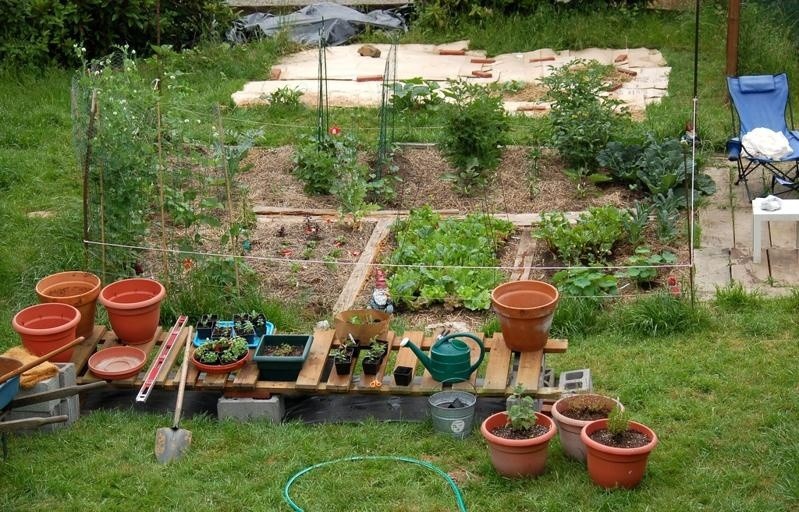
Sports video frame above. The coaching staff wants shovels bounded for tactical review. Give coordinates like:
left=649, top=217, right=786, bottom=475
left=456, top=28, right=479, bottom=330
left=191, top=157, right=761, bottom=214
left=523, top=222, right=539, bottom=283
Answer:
left=154, top=325, right=193, bottom=466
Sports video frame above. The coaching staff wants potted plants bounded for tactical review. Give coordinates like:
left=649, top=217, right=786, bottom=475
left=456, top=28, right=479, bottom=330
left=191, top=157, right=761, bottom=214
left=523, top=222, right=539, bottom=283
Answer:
left=479, top=381, right=558, bottom=479
left=190, top=335, right=250, bottom=374
left=552, top=382, right=625, bottom=462
left=580, top=405, right=658, bottom=489
left=253, top=333, right=314, bottom=381
left=195, top=312, right=266, bottom=344
left=333, top=336, right=387, bottom=375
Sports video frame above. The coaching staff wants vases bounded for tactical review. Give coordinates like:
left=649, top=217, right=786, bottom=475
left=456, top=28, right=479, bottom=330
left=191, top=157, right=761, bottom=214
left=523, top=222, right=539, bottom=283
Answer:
left=490, top=279, right=559, bottom=352
left=97, top=278, right=166, bottom=346
left=11, top=303, right=81, bottom=363
left=36, top=270, right=102, bottom=342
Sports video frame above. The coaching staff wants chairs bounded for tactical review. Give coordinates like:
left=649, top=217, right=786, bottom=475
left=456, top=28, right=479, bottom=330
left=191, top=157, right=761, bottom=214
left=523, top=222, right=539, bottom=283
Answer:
left=726, top=72, right=799, bottom=203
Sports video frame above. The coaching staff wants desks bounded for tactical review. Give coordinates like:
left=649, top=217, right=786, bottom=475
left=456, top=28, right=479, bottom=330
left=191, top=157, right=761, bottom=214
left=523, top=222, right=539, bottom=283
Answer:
left=751, top=198, right=799, bottom=264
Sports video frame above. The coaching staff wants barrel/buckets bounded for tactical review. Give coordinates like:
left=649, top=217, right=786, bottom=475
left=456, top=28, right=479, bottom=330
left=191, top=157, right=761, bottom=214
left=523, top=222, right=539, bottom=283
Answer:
left=427, top=378, right=477, bottom=441
